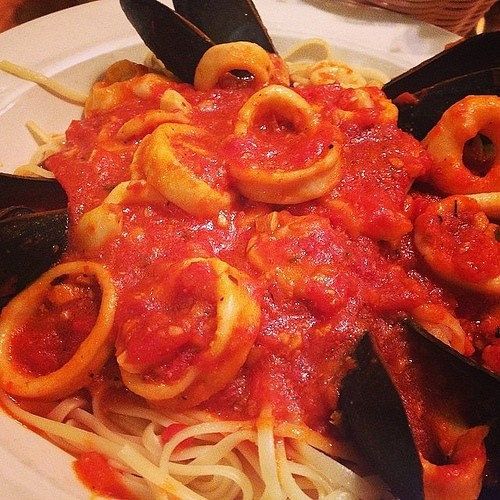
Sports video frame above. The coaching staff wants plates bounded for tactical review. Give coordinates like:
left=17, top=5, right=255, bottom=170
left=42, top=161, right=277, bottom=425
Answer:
left=0, top=0, right=500, bottom=500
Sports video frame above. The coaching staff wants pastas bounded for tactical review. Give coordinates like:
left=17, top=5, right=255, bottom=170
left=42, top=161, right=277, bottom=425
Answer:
left=0, top=36, right=500, bottom=500
